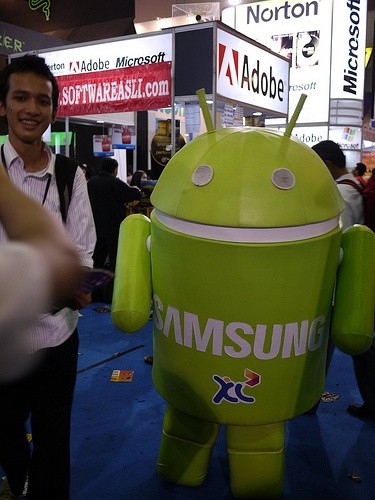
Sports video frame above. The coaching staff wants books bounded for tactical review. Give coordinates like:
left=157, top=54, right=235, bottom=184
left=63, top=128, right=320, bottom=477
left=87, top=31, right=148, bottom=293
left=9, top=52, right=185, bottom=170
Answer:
left=48, top=266, right=115, bottom=315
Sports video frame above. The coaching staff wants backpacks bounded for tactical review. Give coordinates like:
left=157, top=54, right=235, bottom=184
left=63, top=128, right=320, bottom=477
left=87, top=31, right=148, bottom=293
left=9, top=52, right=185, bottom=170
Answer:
left=337, top=179, right=375, bottom=233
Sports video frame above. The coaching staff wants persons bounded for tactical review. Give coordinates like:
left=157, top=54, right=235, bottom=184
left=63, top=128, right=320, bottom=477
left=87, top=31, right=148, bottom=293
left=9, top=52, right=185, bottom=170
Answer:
left=0, top=54, right=97, bottom=500
left=0, top=160, right=83, bottom=341
left=80, top=155, right=153, bottom=268
left=303, top=140, right=375, bottom=420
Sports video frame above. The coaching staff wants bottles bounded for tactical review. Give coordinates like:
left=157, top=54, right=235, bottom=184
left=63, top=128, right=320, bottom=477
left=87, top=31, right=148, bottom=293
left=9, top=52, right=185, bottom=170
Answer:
left=102, top=137, right=110, bottom=152
left=122, top=127, right=131, bottom=144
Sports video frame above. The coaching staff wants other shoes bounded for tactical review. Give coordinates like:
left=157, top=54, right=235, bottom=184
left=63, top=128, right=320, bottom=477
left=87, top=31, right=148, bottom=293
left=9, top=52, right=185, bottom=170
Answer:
left=143, top=355, right=153, bottom=365
left=348, top=403, right=375, bottom=420
